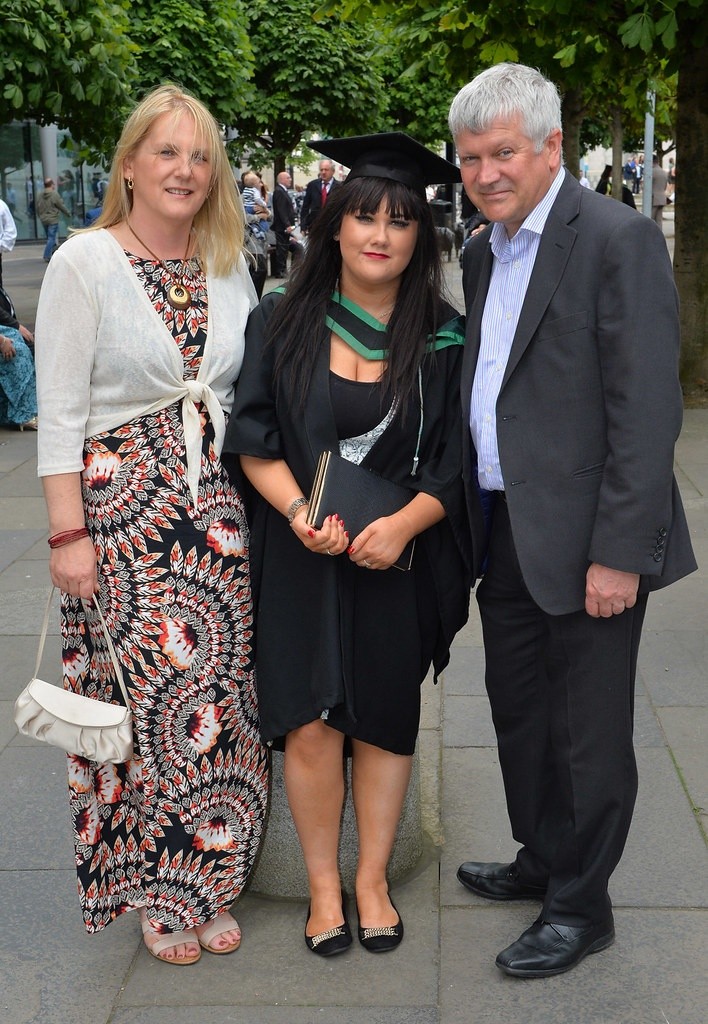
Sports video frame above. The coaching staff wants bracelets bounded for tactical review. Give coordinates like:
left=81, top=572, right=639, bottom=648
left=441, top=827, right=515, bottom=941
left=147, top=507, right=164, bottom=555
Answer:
left=287, top=498, right=310, bottom=523
left=48, top=526, right=91, bottom=550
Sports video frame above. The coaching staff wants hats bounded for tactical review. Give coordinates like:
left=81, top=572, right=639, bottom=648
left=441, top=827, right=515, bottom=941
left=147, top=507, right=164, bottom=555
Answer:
left=306, top=131, right=463, bottom=200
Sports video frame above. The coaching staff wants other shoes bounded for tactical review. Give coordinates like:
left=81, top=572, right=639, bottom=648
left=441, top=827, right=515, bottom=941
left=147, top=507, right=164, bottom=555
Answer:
left=275, top=273, right=287, bottom=278
left=355, top=892, right=404, bottom=953
left=44, top=257, right=51, bottom=263
left=304, top=888, right=353, bottom=957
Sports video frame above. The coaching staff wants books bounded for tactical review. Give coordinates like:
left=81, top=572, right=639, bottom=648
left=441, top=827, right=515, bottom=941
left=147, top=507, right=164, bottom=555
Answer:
left=305, top=451, right=417, bottom=574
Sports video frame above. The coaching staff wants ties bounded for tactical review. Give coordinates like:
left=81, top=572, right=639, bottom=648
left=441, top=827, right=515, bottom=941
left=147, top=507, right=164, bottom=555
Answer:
left=321, top=183, right=329, bottom=209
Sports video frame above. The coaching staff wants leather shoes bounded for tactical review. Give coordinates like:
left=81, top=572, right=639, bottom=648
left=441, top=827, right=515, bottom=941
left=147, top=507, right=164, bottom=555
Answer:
left=495, top=916, right=615, bottom=977
left=456, top=861, right=548, bottom=901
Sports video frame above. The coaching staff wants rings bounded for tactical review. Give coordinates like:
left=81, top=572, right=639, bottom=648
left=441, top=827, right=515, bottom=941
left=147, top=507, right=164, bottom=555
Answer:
left=326, top=549, right=335, bottom=556
left=363, top=559, right=371, bottom=567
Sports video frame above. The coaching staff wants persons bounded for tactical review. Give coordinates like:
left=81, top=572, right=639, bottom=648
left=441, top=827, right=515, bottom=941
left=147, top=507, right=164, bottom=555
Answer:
left=462, top=187, right=490, bottom=252
left=238, top=160, right=343, bottom=302
left=0, top=175, right=34, bottom=431
left=578, top=151, right=675, bottom=233
left=219, top=132, right=476, bottom=959
left=446, top=62, right=699, bottom=981
left=35, top=85, right=274, bottom=966
left=36, top=167, right=106, bottom=263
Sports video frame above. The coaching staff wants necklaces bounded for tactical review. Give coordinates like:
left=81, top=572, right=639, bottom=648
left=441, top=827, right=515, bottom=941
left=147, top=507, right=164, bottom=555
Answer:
left=358, top=306, right=394, bottom=321
left=122, top=220, right=198, bottom=310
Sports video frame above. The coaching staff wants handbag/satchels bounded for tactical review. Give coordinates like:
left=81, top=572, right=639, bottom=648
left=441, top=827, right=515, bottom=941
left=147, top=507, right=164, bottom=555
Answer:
left=14, top=585, right=134, bottom=764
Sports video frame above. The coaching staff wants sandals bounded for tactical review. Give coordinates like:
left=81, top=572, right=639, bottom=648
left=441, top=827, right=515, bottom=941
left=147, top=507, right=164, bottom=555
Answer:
left=142, top=911, right=242, bottom=965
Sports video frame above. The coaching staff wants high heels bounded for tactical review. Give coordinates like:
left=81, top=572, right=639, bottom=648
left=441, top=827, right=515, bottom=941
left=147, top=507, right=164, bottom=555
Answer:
left=19, top=417, right=37, bottom=431
